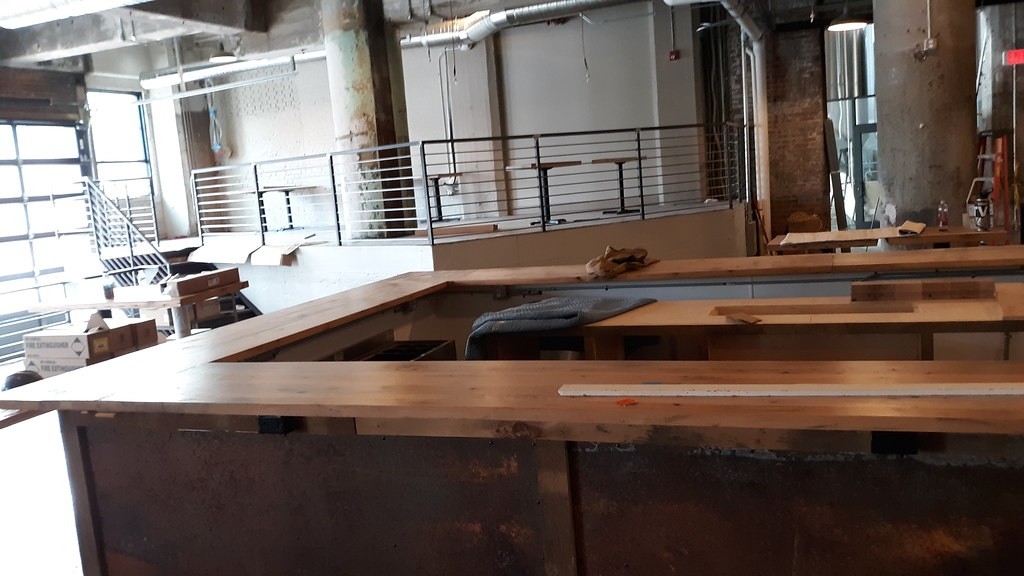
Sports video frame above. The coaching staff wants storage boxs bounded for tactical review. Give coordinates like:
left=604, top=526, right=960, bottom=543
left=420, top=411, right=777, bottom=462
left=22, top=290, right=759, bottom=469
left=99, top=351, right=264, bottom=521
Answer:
left=22, top=295, right=222, bottom=378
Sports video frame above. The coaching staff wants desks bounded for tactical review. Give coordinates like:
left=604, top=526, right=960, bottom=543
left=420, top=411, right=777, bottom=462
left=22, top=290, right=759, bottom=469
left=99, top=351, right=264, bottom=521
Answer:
left=591, top=155, right=647, bottom=215
left=29, top=279, right=250, bottom=347
left=506, top=160, right=582, bottom=225
left=263, top=185, right=316, bottom=231
left=423, top=173, right=462, bottom=221
left=767, top=226, right=1007, bottom=254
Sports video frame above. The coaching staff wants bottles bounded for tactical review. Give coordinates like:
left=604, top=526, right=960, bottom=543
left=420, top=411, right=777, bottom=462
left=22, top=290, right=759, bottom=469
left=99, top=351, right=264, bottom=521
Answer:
left=937, top=200, right=950, bottom=231
left=974, top=187, right=994, bottom=232
